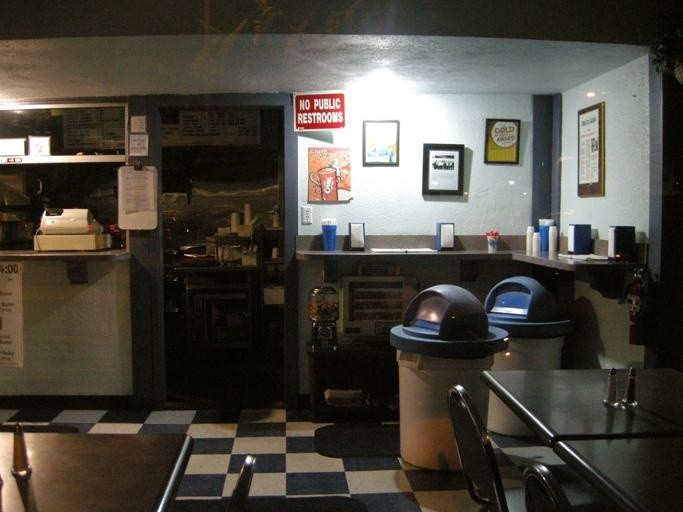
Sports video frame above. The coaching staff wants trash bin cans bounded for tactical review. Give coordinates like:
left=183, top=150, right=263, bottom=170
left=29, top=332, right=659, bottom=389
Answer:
left=390, top=285, right=509, bottom=472
left=484, top=274, right=574, bottom=440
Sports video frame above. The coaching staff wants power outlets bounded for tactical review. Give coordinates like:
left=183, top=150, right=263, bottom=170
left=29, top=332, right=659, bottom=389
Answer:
left=300, top=205, right=312, bottom=224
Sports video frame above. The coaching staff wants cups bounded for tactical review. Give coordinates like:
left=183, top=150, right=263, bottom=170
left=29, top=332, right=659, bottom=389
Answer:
left=538, top=224, right=548, bottom=251
left=487, top=236, right=498, bottom=250
left=310, top=167, right=338, bottom=200
left=322, top=224, right=337, bottom=251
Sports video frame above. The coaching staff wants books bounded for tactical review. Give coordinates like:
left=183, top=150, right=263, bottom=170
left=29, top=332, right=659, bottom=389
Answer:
left=556, top=252, right=608, bottom=261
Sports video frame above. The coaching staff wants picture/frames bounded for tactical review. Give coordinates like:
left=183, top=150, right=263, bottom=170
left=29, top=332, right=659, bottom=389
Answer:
left=361, top=119, right=520, bottom=196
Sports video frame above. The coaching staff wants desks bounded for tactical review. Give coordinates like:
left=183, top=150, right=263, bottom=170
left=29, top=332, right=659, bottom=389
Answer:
left=481, top=366, right=682, bottom=512
left=0, top=431, right=194, bottom=512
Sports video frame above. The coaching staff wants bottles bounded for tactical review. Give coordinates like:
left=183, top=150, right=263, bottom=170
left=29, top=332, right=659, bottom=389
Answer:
left=271, top=247, right=280, bottom=258
left=243, top=203, right=252, bottom=226
left=525, top=225, right=541, bottom=251
left=231, top=212, right=240, bottom=233
left=272, top=204, right=280, bottom=228
left=602, top=366, right=637, bottom=407
left=547, top=225, right=558, bottom=251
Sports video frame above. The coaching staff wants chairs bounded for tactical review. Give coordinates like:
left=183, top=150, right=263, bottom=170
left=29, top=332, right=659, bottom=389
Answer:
left=446, top=383, right=509, bottom=511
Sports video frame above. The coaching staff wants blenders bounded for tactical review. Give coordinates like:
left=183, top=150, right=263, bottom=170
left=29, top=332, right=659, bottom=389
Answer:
left=308, top=287, right=339, bottom=353
